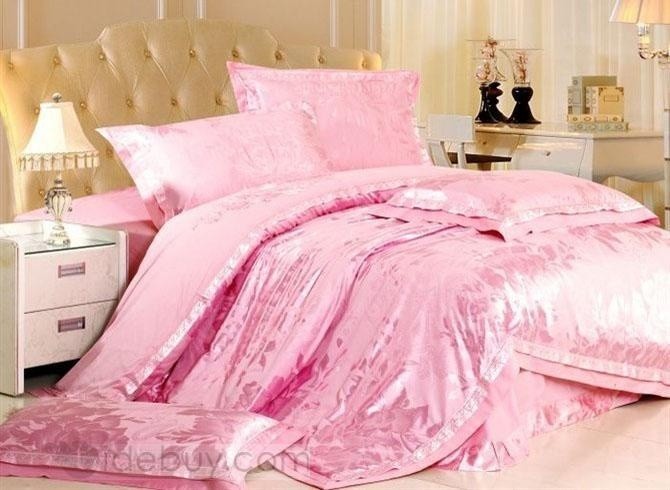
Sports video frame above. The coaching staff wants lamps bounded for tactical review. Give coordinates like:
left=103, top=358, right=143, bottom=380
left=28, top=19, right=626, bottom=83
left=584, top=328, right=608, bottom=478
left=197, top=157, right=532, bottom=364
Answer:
left=609, top=1, right=670, bottom=64
left=17, top=93, right=100, bottom=246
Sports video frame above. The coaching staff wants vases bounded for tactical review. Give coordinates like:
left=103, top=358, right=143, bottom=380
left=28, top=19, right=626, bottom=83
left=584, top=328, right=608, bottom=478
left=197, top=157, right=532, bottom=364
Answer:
left=465, top=33, right=545, bottom=124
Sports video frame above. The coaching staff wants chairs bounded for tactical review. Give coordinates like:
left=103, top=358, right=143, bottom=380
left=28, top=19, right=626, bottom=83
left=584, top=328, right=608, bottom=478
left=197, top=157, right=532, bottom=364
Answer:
left=426, top=114, right=512, bottom=170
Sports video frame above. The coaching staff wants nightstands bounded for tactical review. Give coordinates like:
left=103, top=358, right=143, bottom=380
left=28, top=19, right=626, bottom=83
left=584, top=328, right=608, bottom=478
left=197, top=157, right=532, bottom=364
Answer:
left=0, top=221, right=129, bottom=396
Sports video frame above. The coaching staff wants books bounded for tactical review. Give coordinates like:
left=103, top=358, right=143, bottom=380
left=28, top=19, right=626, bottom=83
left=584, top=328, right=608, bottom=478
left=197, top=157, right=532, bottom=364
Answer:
left=566, top=74, right=630, bottom=134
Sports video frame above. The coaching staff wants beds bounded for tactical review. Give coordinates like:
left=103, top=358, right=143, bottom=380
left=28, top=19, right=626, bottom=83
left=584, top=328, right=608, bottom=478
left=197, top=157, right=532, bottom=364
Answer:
left=1, top=19, right=670, bottom=471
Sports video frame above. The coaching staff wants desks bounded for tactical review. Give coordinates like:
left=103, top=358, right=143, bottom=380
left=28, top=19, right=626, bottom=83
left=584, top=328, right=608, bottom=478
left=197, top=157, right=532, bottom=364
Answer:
left=447, top=124, right=665, bottom=230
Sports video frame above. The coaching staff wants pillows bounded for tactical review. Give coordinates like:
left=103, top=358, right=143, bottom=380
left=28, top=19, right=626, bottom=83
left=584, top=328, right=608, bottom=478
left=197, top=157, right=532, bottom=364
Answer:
left=226, top=61, right=435, bottom=172
left=0, top=399, right=305, bottom=490
left=94, top=108, right=339, bottom=230
left=367, top=170, right=661, bottom=243
